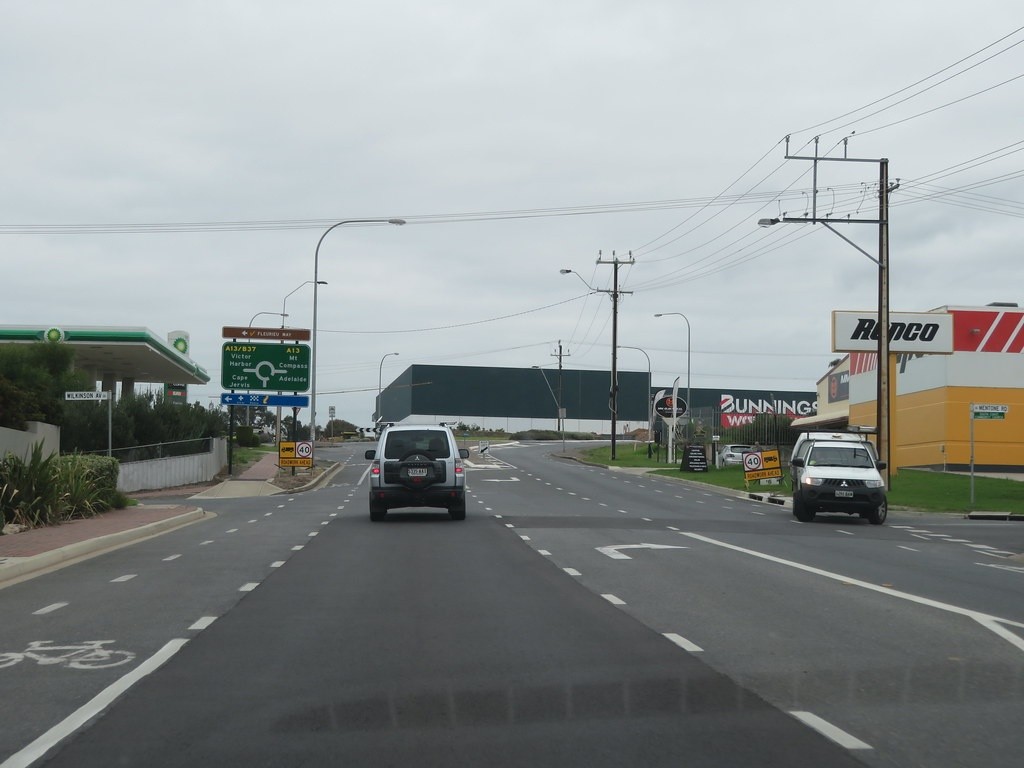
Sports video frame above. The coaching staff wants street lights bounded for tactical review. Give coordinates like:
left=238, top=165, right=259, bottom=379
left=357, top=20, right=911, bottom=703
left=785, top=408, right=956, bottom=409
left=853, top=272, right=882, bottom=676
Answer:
left=246, top=311, right=290, bottom=341
left=282, top=280, right=328, bottom=325
left=378, top=353, right=400, bottom=421
left=757, top=216, right=892, bottom=503
left=616, top=345, right=652, bottom=441
left=654, top=312, right=691, bottom=443
left=560, top=269, right=619, bottom=460
left=308, top=219, right=407, bottom=443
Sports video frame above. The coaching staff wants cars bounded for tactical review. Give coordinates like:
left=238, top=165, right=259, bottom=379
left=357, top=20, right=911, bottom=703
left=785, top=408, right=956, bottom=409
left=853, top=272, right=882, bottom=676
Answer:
left=718, top=444, right=755, bottom=468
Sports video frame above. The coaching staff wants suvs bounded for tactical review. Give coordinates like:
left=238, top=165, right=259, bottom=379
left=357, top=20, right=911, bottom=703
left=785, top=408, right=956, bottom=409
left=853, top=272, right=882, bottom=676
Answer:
left=364, top=423, right=470, bottom=521
left=787, top=430, right=888, bottom=526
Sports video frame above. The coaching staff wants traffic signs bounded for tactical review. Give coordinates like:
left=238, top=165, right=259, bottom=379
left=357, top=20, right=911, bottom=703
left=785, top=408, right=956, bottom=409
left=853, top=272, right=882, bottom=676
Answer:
left=221, top=342, right=310, bottom=391
left=220, top=392, right=310, bottom=407
left=222, top=326, right=311, bottom=341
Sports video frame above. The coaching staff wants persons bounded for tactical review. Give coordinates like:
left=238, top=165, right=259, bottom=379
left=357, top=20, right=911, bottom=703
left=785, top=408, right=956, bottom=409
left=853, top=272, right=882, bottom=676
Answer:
left=810, top=450, right=832, bottom=465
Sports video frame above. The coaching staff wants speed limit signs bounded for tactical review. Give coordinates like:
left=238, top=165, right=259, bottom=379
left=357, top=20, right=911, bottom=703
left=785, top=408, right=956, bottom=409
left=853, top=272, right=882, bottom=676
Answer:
left=296, top=441, right=313, bottom=457
left=742, top=452, right=762, bottom=471
